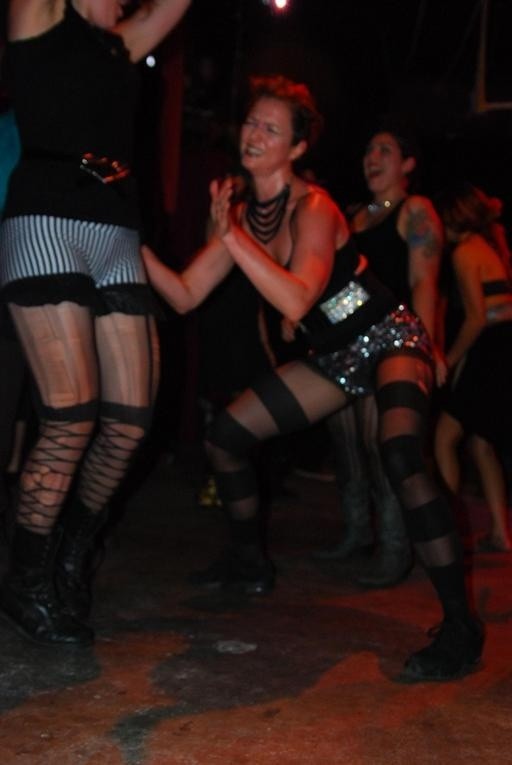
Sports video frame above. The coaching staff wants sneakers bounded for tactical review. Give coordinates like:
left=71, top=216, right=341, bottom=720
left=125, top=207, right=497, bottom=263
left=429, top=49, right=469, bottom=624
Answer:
left=188, top=554, right=275, bottom=594
left=405, top=616, right=484, bottom=680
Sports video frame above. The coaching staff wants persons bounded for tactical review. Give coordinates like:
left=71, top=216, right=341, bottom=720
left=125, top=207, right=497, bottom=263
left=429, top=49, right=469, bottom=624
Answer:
left=278, top=120, right=445, bottom=593
left=137, top=72, right=488, bottom=684
left=0, top=0, right=193, bottom=653
left=432, top=178, right=512, bottom=559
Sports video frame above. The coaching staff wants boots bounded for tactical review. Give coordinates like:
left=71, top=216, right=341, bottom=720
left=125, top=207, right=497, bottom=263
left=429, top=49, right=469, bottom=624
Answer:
left=340, top=474, right=413, bottom=590
left=0, top=499, right=110, bottom=649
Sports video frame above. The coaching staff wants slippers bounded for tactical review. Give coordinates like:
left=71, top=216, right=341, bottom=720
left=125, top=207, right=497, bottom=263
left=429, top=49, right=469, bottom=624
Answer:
left=460, top=536, right=512, bottom=555
